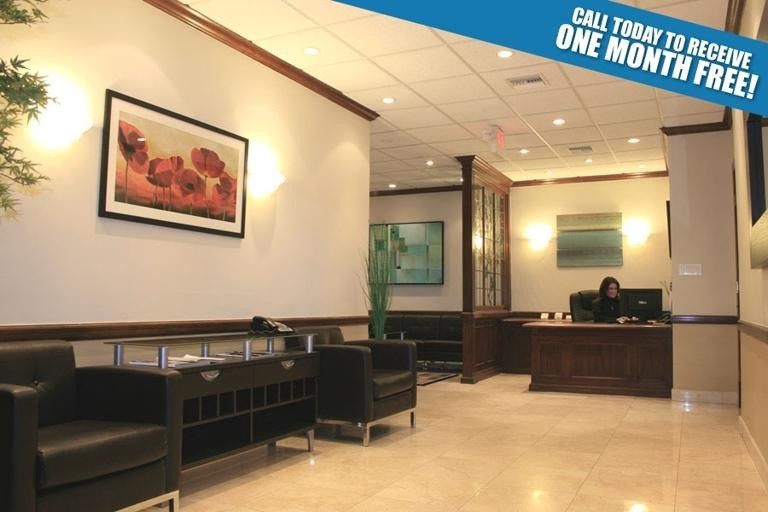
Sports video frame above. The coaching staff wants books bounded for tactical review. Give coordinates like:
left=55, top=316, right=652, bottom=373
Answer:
left=128, top=351, right=283, bottom=369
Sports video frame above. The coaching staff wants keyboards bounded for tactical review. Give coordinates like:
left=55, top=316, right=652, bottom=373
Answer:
left=608, top=320, right=639, bottom=323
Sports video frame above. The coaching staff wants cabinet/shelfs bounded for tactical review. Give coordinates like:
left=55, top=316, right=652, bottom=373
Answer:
left=109, top=337, right=324, bottom=510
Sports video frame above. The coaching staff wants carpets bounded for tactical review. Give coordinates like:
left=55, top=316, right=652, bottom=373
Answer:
left=417, top=371, right=458, bottom=386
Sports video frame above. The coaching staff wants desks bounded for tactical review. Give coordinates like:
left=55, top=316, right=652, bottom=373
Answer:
left=521, top=320, right=673, bottom=397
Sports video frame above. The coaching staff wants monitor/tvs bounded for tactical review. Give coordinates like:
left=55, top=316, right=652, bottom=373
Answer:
left=619, top=289, right=662, bottom=324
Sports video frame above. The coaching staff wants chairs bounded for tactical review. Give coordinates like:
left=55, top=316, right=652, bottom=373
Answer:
left=287, top=325, right=419, bottom=447
left=0, top=342, right=183, bottom=512
left=570, top=289, right=601, bottom=324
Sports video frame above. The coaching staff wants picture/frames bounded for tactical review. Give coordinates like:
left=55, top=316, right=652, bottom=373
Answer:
left=97, top=90, right=253, bottom=239
left=369, top=222, right=445, bottom=287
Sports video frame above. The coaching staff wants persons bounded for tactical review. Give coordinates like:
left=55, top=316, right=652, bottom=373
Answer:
left=592, top=277, right=639, bottom=324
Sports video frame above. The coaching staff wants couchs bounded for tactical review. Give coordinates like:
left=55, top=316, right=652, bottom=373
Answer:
left=370, top=313, right=464, bottom=371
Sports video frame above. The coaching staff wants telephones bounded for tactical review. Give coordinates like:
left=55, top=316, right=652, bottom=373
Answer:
left=250, top=316, right=294, bottom=335
left=657, top=312, right=671, bottom=324
